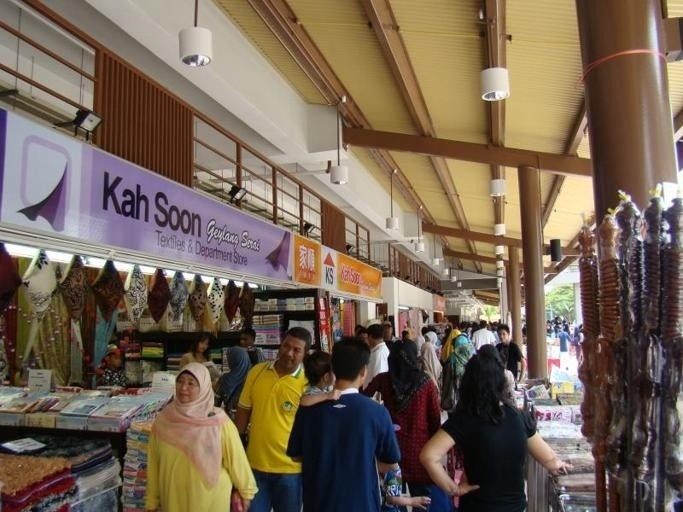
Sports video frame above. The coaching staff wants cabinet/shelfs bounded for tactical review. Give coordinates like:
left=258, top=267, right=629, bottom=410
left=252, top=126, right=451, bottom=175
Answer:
left=440, top=293, right=443, bottom=296
left=340, top=298, right=359, bottom=338
left=119, top=287, right=334, bottom=396
left=0, top=383, right=163, bottom=512
left=512, top=376, right=595, bottom=512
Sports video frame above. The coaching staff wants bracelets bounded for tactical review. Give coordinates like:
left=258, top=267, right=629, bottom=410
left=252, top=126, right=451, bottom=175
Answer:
left=447, top=485, right=458, bottom=495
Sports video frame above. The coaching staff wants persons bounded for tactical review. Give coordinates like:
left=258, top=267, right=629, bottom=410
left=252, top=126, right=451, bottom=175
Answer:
left=494, top=325, right=525, bottom=391
left=574, top=324, right=585, bottom=365
left=379, top=464, right=431, bottom=512
left=420, top=321, right=479, bottom=339
left=235, top=327, right=311, bottom=512
left=298, top=350, right=341, bottom=406
left=417, top=343, right=443, bottom=410
left=471, top=320, right=497, bottom=355
left=360, top=339, right=442, bottom=512
left=487, top=319, right=503, bottom=331
left=358, top=328, right=369, bottom=344
left=237, top=328, right=267, bottom=368
left=180, top=335, right=210, bottom=371
left=544, top=317, right=570, bottom=336
left=213, top=347, right=253, bottom=421
left=400, top=330, right=419, bottom=355
left=442, top=329, right=462, bottom=363
left=362, top=324, right=390, bottom=391
left=354, top=324, right=363, bottom=334
left=420, top=356, right=575, bottom=512
left=83, top=349, right=130, bottom=386
left=440, top=334, right=475, bottom=418
left=286, top=336, right=401, bottom=512
left=381, top=320, right=400, bottom=350
left=425, top=332, right=440, bottom=359
left=559, top=329, right=572, bottom=370
left=144, top=363, right=260, bottom=512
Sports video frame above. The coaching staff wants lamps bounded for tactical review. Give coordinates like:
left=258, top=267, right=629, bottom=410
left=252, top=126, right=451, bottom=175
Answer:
left=437, top=291, right=441, bottom=294
left=347, top=244, right=352, bottom=252
left=229, top=186, right=247, bottom=201
left=494, top=197, right=505, bottom=235
left=497, top=283, right=502, bottom=288
left=432, top=233, right=440, bottom=265
left=386, top=169, right=398, bottom=229
left=303, top=223, right=315, bottom=234
left=405, top=275, right=411, bottom=280
left=496, top=255, right=504, bottom=269
left=415, top=281, right=420, bottom=284
left=330, top=102, right=349, bottom=184
left=413, top=203, right=425, bottom=252
left=432, top=290, right=437, bottom=293
left=179, top=0, right=214, bottom=67
left=75, top=110, right=103, bottom=132
left=443, top=253, right=449, bottom=276
left=497, top=277, right=503, bottom=282
left=427, top=286, right=432, bottom=291
left=457, top=274, right=462, bottom=288
left=451, top=265, right=456, bottom=281
left=479, top=18, right=509, bottom=100
left=496, top=236, right=504, bottom=254
left=496, top=269, right=504, bottom=276
left=491, top=140, right=506, bottom=196
